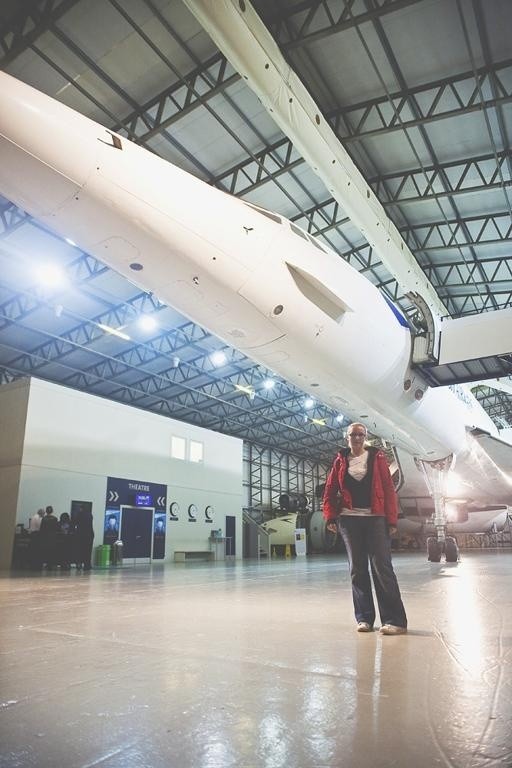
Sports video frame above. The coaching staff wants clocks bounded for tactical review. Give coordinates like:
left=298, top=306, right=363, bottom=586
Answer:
left=188, top=503, right=197, bottom=518
left=205, top=505, right=215, bottom=519
left=169, top=502, right=181, bottom=516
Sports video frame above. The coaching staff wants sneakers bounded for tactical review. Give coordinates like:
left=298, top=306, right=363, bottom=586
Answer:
left=378, top=623, right=407, bottom=635
left=356, top=620, right=373, bottom=632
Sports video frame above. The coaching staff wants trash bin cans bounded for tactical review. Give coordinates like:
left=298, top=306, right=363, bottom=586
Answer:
left=97, top=544, right=111, bottom=568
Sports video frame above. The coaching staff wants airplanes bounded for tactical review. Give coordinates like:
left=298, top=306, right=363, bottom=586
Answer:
left=0, top=73, right=512, bottom=564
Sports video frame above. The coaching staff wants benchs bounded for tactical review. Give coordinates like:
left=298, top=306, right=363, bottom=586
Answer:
left=174, top=550, right=216, bottom=562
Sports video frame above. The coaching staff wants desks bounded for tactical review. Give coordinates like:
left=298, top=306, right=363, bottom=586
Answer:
left=209, top=536, right=233, bottom=561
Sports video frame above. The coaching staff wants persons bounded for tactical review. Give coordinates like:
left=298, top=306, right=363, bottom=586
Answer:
left=323, top=422, right=408, bottom=635
left=103, top=514, right=120, bottom=545
left=151, top=518, right=167, bottom=559
left=26, top=504, right=95, bottom=569
